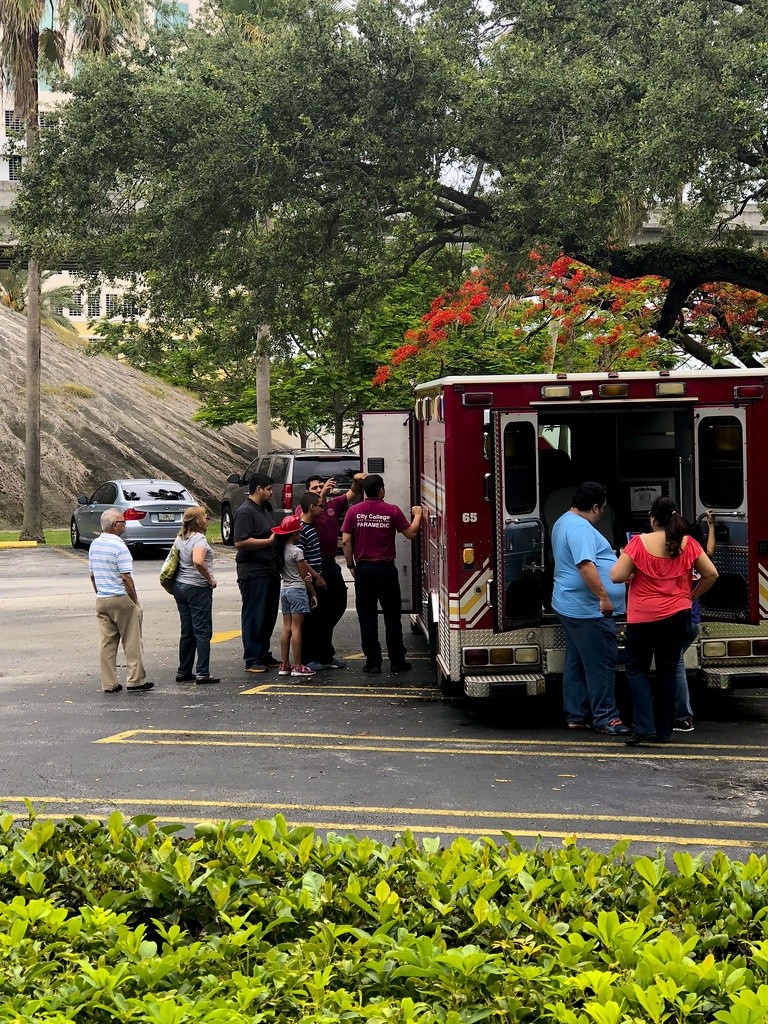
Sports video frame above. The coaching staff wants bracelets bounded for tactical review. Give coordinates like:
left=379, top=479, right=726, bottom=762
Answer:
left=347, top=562, right=355, bottom=569
left=316, top=574, right=318, bottom=578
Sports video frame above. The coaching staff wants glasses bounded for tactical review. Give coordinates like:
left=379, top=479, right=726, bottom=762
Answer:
left=111, top=521, right=126, bottom=526
left=205, top=516, right=210, bottom=520
left=309, top=504, right=325, bottom=509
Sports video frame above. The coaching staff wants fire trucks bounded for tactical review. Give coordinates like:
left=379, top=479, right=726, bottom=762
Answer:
left=357, top=367, right=768, bottom=719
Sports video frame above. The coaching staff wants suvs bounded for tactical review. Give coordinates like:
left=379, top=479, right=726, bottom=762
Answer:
left=220, top=448, right=361, bottom=545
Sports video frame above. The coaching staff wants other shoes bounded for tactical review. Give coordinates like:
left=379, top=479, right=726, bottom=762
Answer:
left=306, top=658, right=347, bottom=670
left=244, top=663, right=267, bottom=672
left=624, top=733, right=656, bottom=745
left=127, top=681, right=154, bottom=691
left=105, top=684, right=123, bottom=693
left=362, top=664, right=382, bottom=674
left=175, top=673, right=196, bottom=682
left=267, top=660, right=283, bottom=666
left=196, top=675, right=220, bottom=684
left=390, top=662, right=413, bottom=672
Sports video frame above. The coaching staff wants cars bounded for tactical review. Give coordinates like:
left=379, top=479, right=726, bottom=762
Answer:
left=70, top=478, right=199, bottom=555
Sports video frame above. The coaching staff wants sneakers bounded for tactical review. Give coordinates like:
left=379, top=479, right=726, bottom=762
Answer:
left=278, top=664, right=291, bottom=675
left=568, top=717, right=593, bottom=729
left=290, top=664, right=316, bottom=676
left=672, top=719, right=694, bottom=732
left=597, top=717, right=630, bottom=734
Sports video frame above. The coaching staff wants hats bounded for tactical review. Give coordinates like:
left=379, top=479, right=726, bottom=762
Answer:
left=271, top=516, right=304, bottom=534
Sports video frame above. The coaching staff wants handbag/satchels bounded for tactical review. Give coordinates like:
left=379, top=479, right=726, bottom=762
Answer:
left=160, top=547, right=178, bottom=594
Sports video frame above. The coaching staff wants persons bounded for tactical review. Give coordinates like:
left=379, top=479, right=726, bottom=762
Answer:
left=296, top=492, right=328, bottom=672
left=163, top=507, right=224, bottom=685
left=340, top=475, right=421, bottom=673
left=550, top=482, right=631, bottom=737
left=271, top=515, right=319, bottom=677
left=669, top=509, right=718, bottom=737
left=295, top=471, right=368, bottom=672
left=233, top=473, right=284, bottom=674
left=611, top=496, right=717, bottom=747
left=88, top=508, right=155, bottom=694
